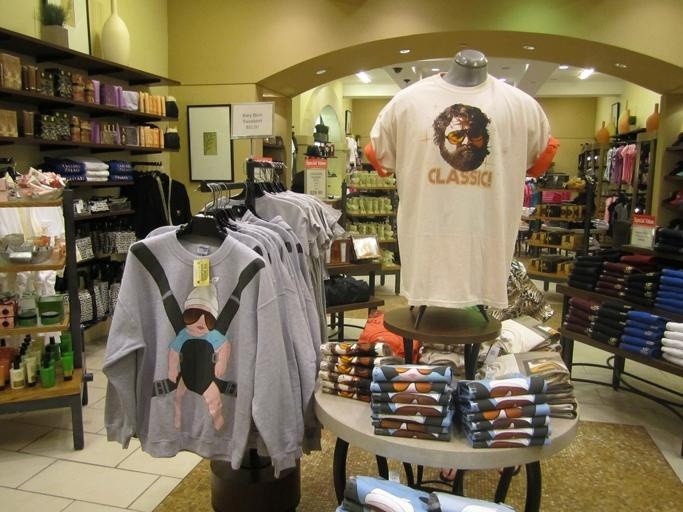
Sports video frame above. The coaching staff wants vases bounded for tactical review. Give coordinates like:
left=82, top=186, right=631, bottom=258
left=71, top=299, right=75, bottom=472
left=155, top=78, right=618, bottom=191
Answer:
left=645, top=103, right=660, bottom=134
left=596, top=121, right=609, bottom=143
left=100, top=1, right=132, bottom=67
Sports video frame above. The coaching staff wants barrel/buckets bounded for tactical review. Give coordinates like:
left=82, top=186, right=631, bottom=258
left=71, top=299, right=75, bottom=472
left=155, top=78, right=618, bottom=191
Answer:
left=116, top=121, right=121, bottom=144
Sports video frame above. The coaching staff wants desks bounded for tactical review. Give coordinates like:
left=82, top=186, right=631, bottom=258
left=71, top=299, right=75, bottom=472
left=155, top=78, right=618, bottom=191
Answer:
left=310, top=352, right=581, bottom=511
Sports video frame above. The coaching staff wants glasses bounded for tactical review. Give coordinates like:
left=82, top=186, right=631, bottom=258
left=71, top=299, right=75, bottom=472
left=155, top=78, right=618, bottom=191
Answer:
left=182, top=309, right=216, bottom=331
left=444, top=125, right=488, bottom=146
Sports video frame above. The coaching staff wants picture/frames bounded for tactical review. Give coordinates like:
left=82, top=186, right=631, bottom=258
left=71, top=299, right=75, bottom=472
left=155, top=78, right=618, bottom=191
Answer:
left=186, top=103, right=235, bottom=184
left=611, top=102, right=619, bottom=134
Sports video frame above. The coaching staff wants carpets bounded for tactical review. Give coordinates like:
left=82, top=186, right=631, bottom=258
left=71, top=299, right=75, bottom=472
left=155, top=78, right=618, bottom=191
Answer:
left=151, top=421, right=682, bottom=511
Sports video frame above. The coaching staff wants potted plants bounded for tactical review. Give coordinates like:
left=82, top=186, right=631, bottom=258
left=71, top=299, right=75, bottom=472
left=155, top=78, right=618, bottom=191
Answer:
left=40, top=4, right=69, bottom=48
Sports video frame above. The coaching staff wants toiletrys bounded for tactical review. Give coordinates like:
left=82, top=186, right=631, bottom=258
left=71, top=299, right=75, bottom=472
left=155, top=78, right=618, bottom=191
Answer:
left=92, top=79, right=101, bottom=104
left=21, top=63, right=40, bottom=91
left=60, top=330, right=75, bottom=381
left=95, top=122, right=101, bottom=144
left=113, top=85, right=127, bottom=107
left=139, top=91, right=167, bottom=150
left=0, top=332, right=60, bottom=389
left=22, top=108, right=35, bottom=136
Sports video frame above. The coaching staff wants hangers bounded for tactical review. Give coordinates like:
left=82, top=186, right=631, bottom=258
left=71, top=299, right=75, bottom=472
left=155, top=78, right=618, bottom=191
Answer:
left=177, top=161, right=287, bottom=241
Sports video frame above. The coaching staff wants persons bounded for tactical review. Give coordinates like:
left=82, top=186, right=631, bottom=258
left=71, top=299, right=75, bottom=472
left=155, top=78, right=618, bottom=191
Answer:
left=435, top=103, right=490, bottom=172
left=166, top=283, right=230, bottom=432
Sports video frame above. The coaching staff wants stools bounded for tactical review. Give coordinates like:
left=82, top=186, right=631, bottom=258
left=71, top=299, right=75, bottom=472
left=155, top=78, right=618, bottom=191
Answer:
left=382, top=307, right=504, bottom=381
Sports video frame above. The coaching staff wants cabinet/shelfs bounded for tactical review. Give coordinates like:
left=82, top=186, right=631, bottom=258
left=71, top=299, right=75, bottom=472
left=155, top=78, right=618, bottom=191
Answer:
left=1, top=186, right=95, bottom=450
left=514, top=127, right=683, bottom=449
left=1, top=29, right=184, bottom=342
left=325, top=181, right=403, bottom=344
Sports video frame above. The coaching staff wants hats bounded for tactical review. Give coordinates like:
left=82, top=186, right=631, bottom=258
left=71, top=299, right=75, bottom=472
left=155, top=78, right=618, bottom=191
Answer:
left=183, top=283, right=218, bottom=320
